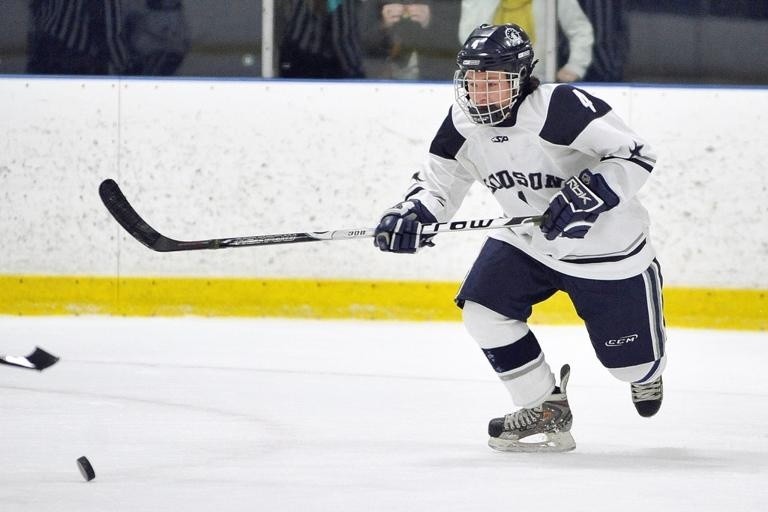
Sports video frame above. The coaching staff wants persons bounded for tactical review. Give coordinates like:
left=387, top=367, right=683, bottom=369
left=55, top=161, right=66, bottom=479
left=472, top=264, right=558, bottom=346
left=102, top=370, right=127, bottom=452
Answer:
left=274, top=1, right=362, bottom=82
left=372, top=22, right=666, bottom=442
left=557, top=1, right=637, bottom=86
left=457, top=1, right=591, bottom=84
left=360, top=1, right=460, bottom=77
left=25, top=0, right=193, bottom=77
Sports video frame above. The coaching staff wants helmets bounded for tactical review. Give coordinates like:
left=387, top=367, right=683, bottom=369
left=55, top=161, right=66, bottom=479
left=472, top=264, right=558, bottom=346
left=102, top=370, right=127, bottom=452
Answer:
left=453, top=23, right=540, bottom=127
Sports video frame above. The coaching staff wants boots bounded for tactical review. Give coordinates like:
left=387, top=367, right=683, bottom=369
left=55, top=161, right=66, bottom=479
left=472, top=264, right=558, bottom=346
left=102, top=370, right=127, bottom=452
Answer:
left=631, top=374, right=663, bottom=418
left=488, top=364, right=573, bottom=441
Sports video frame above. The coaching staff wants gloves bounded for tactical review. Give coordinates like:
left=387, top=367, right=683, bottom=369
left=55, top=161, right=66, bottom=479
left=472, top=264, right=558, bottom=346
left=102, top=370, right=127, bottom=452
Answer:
left=373, top=199, right=439, bottom=254
left=540, top=169, right=620, bottom=240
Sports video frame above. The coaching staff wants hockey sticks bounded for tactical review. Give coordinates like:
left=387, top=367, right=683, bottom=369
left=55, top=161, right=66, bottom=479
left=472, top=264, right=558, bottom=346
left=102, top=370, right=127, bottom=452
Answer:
left=96, top=178, right=547, bottom=253
